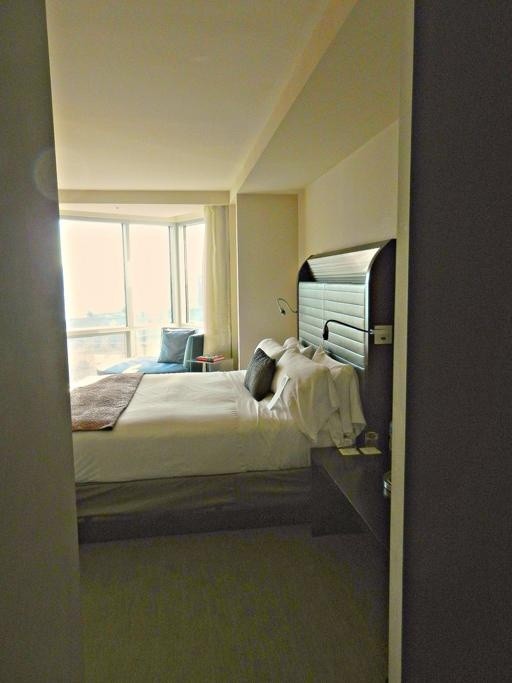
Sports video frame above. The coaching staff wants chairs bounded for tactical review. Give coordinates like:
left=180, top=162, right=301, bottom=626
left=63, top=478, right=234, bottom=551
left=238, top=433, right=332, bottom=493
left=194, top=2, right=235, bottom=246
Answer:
left=96, top=333, right=203, bottom=373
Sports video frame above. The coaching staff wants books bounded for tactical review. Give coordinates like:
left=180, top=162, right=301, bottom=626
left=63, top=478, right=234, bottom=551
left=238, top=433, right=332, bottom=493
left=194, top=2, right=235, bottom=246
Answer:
left=196, top=352, right=225, bottom=361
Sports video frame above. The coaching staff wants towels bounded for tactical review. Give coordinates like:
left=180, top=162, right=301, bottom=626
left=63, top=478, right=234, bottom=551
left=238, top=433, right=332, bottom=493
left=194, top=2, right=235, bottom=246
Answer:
left=69, top=373, right=143, bottom=431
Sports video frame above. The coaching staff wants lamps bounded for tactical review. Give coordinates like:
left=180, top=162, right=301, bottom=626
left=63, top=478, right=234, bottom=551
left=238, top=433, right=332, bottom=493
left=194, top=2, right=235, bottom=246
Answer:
left=276, top=295, right=392, bottom=346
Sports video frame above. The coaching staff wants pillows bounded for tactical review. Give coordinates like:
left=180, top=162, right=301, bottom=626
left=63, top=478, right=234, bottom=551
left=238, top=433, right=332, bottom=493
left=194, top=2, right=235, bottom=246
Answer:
left=156, top=326, right=195, bottom=364
left=243, top=335, right=367, bottom=446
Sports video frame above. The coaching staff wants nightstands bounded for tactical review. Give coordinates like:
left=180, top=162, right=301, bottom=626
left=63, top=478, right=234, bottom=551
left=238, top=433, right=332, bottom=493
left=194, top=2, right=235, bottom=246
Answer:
left=311, top=446, right=389, bottom=552
left=188, top=358, right=233, bottom=372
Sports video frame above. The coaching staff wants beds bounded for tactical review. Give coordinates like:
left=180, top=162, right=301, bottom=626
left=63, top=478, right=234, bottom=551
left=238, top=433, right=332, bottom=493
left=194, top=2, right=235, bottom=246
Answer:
left=68, top=237, right=395, bottom=539
